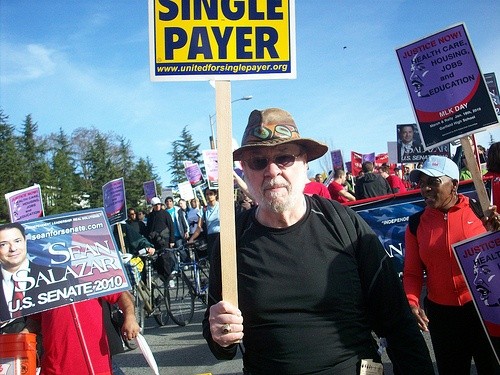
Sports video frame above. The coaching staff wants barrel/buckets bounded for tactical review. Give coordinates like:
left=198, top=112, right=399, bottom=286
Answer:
left=0, top=333, right=38, bottom=375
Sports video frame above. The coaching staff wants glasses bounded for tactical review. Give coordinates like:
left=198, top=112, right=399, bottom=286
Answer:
left=244, top=152, right=301, bottom=171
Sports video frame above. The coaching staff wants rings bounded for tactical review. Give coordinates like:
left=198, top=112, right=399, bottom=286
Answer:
left=494, top=217, right=497, bottom=220
left=221, top=323, right=232, bottom=334
left=123, top=332, right=127, bottom=337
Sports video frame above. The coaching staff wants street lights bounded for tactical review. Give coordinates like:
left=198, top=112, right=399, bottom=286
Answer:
left=209, top=96, right=253, bottom=148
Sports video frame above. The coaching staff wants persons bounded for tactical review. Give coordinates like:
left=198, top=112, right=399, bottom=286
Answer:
left=201, top=112, right=435, bottom=375
left=112, top=187, right=257, bottom=288
left=403, top=155, right=500, bottom=375
left=0, top=222, right=88, bottom=322
left=26, top=292, right=140, bottom=375
left=398, top=125, right=424, bottom=163
left=303, top=142, right=500, bottom=215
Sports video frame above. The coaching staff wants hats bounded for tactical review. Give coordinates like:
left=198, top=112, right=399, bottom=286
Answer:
left=233, top=107, right=329, bottom=163
left=409, top=154, right=460, bottom=183
left=151, top=197, right=162, bottom=205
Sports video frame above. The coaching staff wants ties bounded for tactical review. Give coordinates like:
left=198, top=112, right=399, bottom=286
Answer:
left=11, top=274, right=24, bottom=318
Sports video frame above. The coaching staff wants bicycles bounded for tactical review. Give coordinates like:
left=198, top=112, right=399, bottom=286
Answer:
left=107, top=238, right=211, bottom=355
